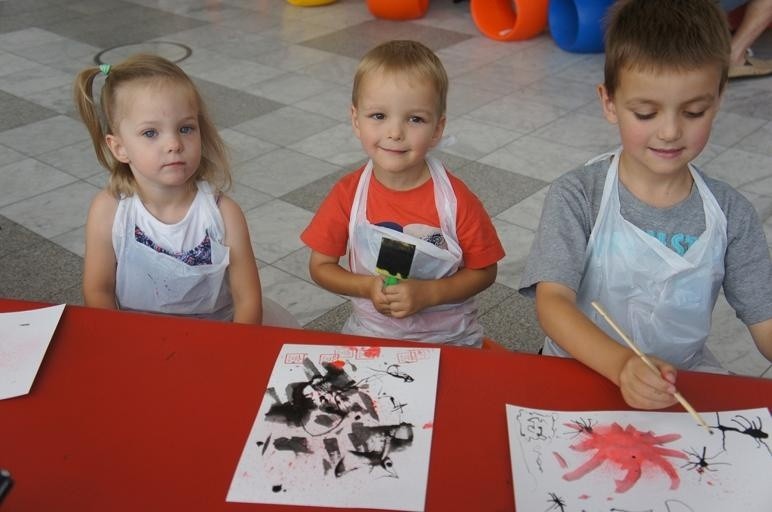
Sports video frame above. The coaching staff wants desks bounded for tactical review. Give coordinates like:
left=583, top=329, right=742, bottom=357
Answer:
left=0, top=296, right=772, bottom=512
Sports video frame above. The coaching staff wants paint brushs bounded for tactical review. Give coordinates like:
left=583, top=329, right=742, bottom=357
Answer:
left=591, top=301, right=714, bottom=435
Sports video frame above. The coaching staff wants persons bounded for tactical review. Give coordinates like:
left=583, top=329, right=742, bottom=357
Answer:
left=515, top=0, right=772, bottom=411
left=296, top=38, right=510, bottom=352
left=72, top=51, right=266, bottom=327
left=726, top=0, right=772, bottom=81
